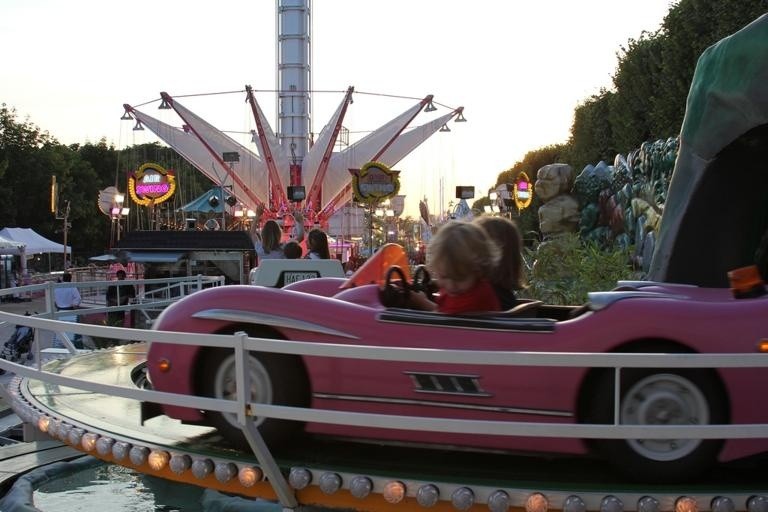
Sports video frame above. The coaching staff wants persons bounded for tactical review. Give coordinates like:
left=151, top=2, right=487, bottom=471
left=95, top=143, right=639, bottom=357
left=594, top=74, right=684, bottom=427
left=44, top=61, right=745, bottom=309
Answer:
left=304, top=229, right=330, bottom=259
left=408, top=220, right=501, bottom=316
left=249, top=202, right=285, bottom=267
left=474, top=215, right=524, bottom=312
left=53, top=272, right=83, bottom=350
left=283, top=240, right=303, bottom=260
left=103, top=270, right=136, bottom=346
left=341, top=247, right=350, bottom=275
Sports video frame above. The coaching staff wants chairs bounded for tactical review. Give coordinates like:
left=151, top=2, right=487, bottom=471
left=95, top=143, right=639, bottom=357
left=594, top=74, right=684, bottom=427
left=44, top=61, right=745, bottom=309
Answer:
left=485, top=295, right=547, bottom=319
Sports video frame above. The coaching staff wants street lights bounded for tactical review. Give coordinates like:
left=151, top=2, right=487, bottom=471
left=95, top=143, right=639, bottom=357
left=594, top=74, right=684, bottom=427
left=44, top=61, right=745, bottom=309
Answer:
left=111, top=192, right=130, bottom=240
